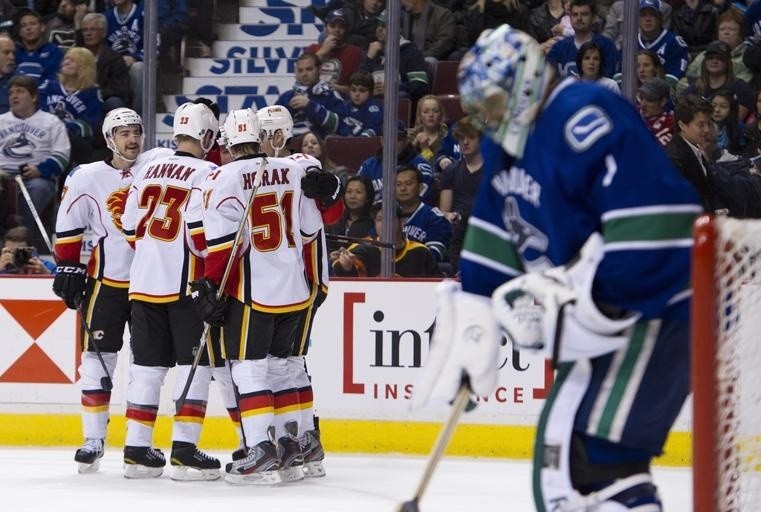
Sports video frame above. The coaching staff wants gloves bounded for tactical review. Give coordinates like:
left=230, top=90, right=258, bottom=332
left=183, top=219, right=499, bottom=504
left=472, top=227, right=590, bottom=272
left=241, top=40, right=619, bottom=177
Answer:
left=301, top=164, right=343, bottom=208
left=188, top=276, right=229, bottom=328
left=52, top=260, right=88, bottom=310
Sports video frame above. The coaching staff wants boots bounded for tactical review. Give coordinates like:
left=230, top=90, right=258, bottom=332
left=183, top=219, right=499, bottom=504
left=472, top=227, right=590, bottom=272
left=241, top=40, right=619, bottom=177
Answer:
left=226, top=415, right=325, bottom=475
left=75, top=418, right=109, bottom=464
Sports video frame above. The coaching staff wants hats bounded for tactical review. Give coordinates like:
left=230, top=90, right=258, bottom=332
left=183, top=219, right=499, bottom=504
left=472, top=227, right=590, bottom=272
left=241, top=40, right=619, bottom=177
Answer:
left=373, top=9, right=388, bottom=24
left=703, top=39, right=730, bottom=59
left=324, top=8, right=349, bottom=27
left=638, top=0, right=659, bottom=15
left=635, top=76, right=670, bottom=101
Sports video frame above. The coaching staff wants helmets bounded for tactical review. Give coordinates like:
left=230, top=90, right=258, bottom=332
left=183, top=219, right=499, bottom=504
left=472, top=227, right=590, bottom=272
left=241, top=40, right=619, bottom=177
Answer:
left=101, top=107, right=145, bottom=152
left=173, top=102, right=294, bottom=149
left=457, top=23, right=555, bottom=159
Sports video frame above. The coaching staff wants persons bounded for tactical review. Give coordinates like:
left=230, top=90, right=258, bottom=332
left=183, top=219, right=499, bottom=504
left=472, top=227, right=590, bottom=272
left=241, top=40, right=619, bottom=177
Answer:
left=53, top=102, right=345, bottom=486
left=1, top=1, right=240, bottom=274
left=406, top=23, right=741, bottom=512
left=274, top=1, right=761, bottom=281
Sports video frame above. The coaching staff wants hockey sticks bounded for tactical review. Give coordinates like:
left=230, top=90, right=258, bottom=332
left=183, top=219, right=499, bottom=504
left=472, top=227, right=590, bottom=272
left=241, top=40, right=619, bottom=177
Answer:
left=156, top=155, right=268, bottom=416
left=14, top=175, right=114, bottom=392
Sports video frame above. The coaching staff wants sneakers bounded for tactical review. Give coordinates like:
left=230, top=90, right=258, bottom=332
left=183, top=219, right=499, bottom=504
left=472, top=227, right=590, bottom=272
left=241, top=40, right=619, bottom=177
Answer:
left=124, top=443, right=167, bottom=467
left=170, top=442, right=221, bottom=469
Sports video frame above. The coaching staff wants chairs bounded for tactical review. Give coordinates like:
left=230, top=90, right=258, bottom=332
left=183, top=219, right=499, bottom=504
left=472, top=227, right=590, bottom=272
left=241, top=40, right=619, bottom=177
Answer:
left=376, top=98, right=411, bottom=132
left=432, top=61, right=462, bottom=95
left=437, top=95, right=464, bottom=126
left=158, top=36, right=186, bottom=82
left=322, top=135, right=383, bottom=184
left=424, top=57, right=438, bottom=95
